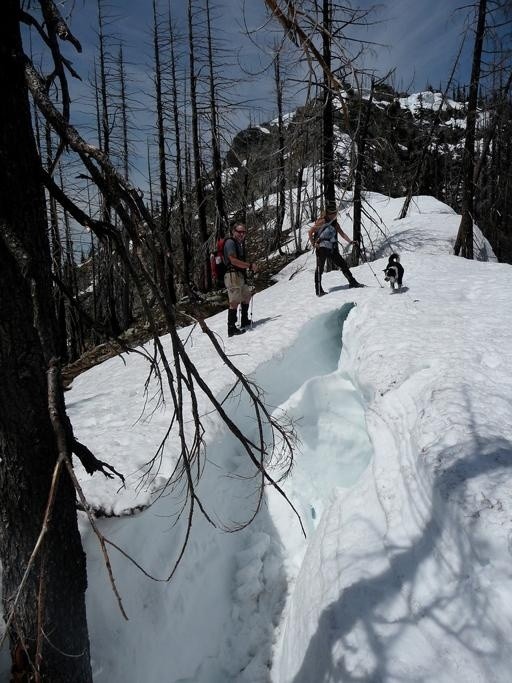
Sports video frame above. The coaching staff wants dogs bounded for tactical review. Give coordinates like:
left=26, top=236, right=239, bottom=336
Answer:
left=382, top=253, right=404, bottom=290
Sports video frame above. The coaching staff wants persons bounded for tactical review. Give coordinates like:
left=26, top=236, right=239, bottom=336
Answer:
left=221, top=220, right=261, bottom=337
left=308, top=204, right=366, bottom=297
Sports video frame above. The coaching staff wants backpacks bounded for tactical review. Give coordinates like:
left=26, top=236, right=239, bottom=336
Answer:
left=210, top=237, right=239, bottom=288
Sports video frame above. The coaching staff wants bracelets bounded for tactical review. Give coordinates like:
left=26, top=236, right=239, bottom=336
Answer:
left=249, top=263, right=252, bottom=269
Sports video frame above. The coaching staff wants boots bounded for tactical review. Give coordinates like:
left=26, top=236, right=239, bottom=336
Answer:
left=315, top=285, right=328, bottom=296
left=349, top=278, right=364, bottom=288
left=228, top=325, right=246, bottom=337
left=241, top=317, right=253, bottom=328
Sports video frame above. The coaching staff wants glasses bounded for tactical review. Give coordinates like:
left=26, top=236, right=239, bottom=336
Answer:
left=328, top=212, right=336, bottom=215
left=234, top=228, right=247, bottom=234
